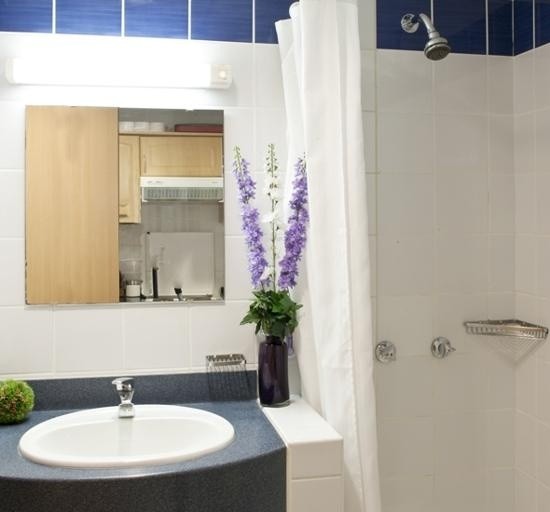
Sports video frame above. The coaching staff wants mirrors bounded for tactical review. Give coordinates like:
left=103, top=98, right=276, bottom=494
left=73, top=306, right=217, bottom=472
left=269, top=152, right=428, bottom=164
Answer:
left=118, top=107, right=227, bottom=303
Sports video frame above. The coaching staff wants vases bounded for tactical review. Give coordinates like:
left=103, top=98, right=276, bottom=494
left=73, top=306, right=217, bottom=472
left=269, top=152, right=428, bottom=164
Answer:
left=256, top=336, right=290, bottom=407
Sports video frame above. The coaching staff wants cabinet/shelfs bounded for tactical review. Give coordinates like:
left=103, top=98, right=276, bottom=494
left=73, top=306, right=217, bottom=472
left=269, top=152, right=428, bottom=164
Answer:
left=117, top=133, right=224, bottom=224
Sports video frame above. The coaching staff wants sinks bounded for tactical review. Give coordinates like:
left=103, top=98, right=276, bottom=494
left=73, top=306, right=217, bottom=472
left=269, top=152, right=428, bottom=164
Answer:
left=18, top=404, right=235, bottom=468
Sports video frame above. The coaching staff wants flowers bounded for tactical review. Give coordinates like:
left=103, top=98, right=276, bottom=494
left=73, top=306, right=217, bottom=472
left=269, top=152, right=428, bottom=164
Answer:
left=234, top=138, right=324, bottom=336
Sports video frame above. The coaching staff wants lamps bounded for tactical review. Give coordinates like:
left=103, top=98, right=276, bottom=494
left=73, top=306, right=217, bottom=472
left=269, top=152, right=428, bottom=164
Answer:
left=10, top=55, right=230, bottom=89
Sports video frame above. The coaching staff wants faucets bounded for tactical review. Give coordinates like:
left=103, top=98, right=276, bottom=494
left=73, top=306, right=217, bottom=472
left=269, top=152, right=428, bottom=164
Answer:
left=112, top=377, right=136, bottom=416
